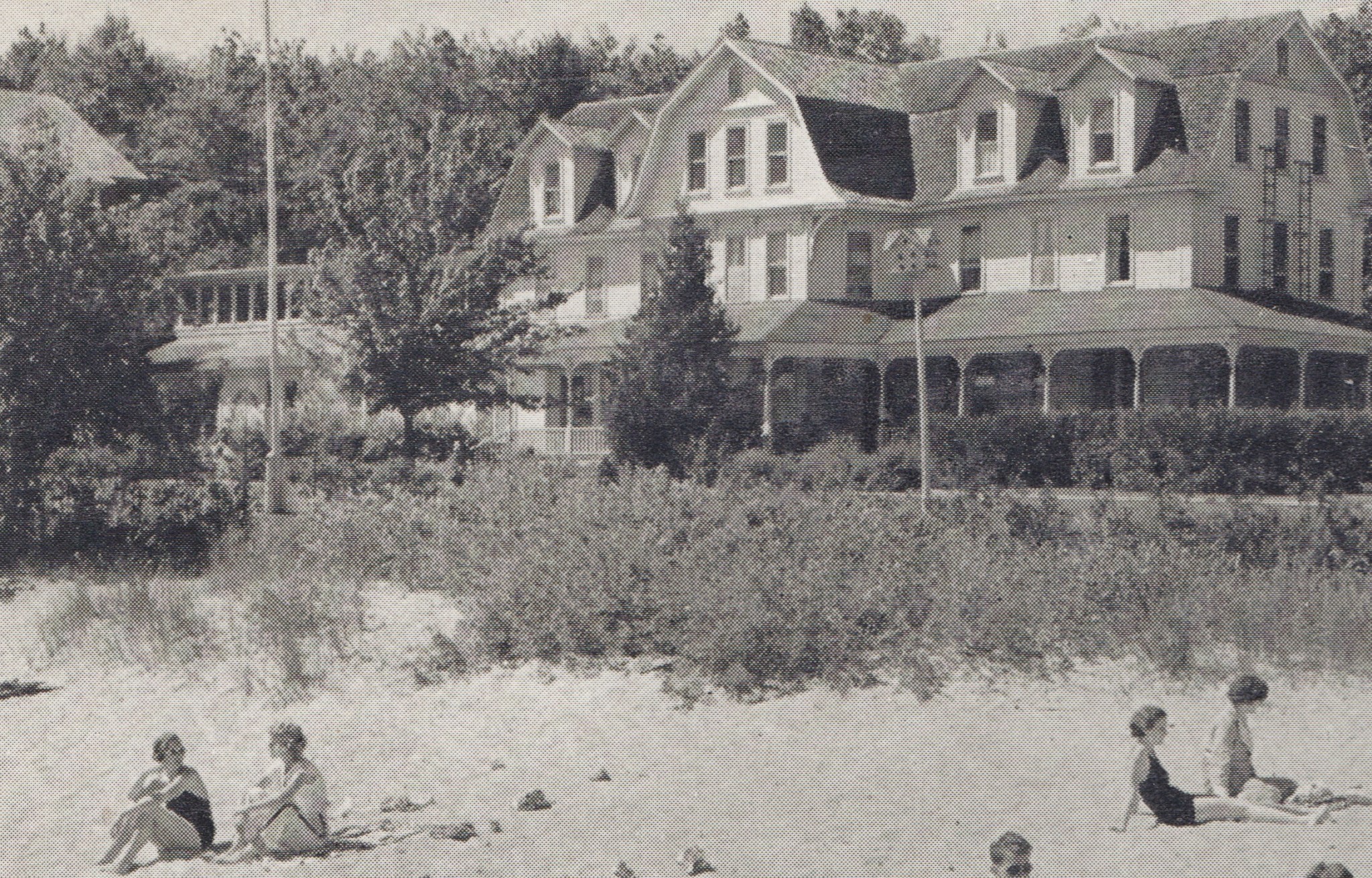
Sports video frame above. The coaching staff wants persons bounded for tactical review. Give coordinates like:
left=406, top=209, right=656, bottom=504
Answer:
left=988, top=830, right=1034, bottom=878
left=1108, top=704, right=1330, bottom=834
left=95, top=731, right=214, bottom=874
left=1205, top=672, right=1372, bottom=809
left=214, top=722, right=328, bottom=863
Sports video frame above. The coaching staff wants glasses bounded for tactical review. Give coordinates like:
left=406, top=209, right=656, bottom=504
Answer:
left=165, top=746, right=185, bottom=754
left=1002, top=863, right=1032, bottom=875
